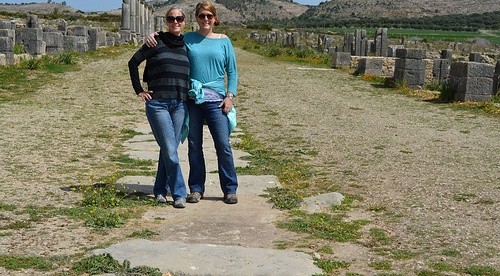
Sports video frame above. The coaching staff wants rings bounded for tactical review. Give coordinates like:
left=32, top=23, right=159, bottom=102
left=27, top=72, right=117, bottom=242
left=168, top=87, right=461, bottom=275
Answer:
left=146, top=39, right=148, bottom=41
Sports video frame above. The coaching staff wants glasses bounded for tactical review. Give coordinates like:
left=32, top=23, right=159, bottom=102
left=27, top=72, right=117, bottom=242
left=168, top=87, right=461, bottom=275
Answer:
left=197, top=14, right=214, bottom=20
left=167, top=16, right=184, bottom=23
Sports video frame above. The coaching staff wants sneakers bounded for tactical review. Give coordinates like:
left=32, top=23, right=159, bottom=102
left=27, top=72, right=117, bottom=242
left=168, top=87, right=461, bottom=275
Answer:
left=173, top=198, right=187, bottom=208
left=224, top=193, right=238, bottom=204
left=156, top=193, right=168, bottom=204
left=188, top=191, right=203, bottom=204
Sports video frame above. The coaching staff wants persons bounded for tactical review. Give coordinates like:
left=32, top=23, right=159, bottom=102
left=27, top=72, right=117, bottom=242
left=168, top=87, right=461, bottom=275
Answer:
left=128, top=5, right=191, bottom=208
left=144, top=1, right=238, bottom=204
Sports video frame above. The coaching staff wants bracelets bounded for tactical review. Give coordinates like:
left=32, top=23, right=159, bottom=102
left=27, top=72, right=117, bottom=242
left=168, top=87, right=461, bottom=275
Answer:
left=227, top=96, right=232, bottom=100
